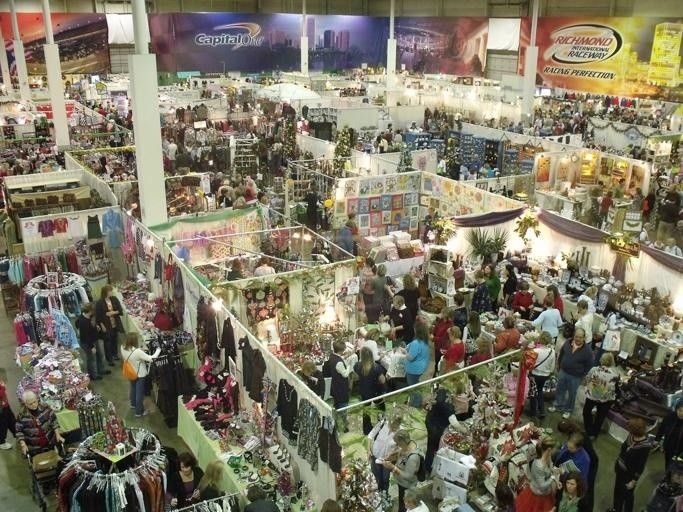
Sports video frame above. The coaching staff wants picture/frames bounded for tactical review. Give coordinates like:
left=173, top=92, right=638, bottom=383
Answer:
left=336, top=175, right=420, bottom=232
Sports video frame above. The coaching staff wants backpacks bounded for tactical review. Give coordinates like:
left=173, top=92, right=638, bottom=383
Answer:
left=122, top=348, right=142, bottom=381
left=402, top=454, right=426, bottom=482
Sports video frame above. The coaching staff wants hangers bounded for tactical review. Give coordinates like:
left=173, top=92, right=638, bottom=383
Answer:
left=67, top=423, right=164, bottom=490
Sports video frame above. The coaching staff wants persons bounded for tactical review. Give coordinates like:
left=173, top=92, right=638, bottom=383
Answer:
left=312, top=239, right=334, bottom=264
left=100, top=286, right=125, bottom=366
left=577, top=286, right=598, bottom=316
left=437, top=326, right=464, bottom=371
left=462, top=311, right=481, bottom=367
left=469, top=270, right=492, bottom=312
left=360, top=258, right=377, bottom=312
left=514, top=434, right=563, bottom=512
left=164, top=453, right=219, bottom=506
left=557, top=420, right=598, bottom=512
left=429, top=306, right=454, bottom=378
left=384, top=115, right=449, bottom=143
left=370, top=264, right=399, bottom=315
left=367, top=413, right=402, bottom=512
left=593, top=297, right=625, bottom=366
left=574, top=300, right=593, bottom=350
left=76, top=299, right=112, bottom=380
left=162, top=137, right=209, bottom=172
left=588, top=177, right=655, bottom=230
left=348, top=212, right=358, bottom=256
left=511, top=281, right=534, bottom=320
left=384, top=275, right=421, bottom=335
left=471, top=337, right=492, bottom=364
left=524, top=294, right=562, bottom=338
left=543, top=285, right=563, bottom=316
left=193, top=461, right=226, bottom=497
left=254, top=257, right=275, bottom=277
left=383, top=428, right=420, bottom=512
left=550, top=473, right=591, bottom=512
left=502, top=265, right=517, bottom=310
left=384, top=296, right=415, bottom=345
left=401, top=323, right=430, bottom=408
left=493, top=316, right=520, bottom=353
left=654, top=401, right=683, bottom=471
left=552, top=432, right=591, bottom=496
left=483, top=264, right=501, bottom=314
left=605, top=419, right=651, bottom=512
left=527, top=331, right=555, bottom=419
left=302, top=182, right=317, bottom=232
left=353, top=347, right=388, bottom=435
left=547, top=327, right=593, bottom=419
left=227, top=257, right=247, bottom=282
left=211, top=169, right=259, bottom=210
left=328, top=341, right=352, bottom=433
left=16, top=390, right=66, bottom=511
left=121, top=332, right=162, bottom=418
left=424, top=388, right=467, bottom=472
left=1, top=369, right=17, bottom=450
left=582, top=352, right=620, bottom=440
left=341, top=219, right=353, bottom=255
left=244, top=485, right=280, bottom=512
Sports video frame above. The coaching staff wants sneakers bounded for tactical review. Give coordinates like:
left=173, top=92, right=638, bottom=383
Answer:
left=128, top=402, right=136, bottom=408
left=99, top=369, right=112, bottom=377
left=563, top=411, right=571, bottom=419
left=134, top=408, right=150, bottom=417
left=0, top=443, right=13, bottom=451
left=89, top=373, right=103, bottom=381
left=547, top=406, right=562, bottom=414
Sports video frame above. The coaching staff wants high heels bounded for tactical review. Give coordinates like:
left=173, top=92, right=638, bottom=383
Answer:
left=107, top=360, right=115, bottom=368
left=113, top=356, right=121, bottom=361
